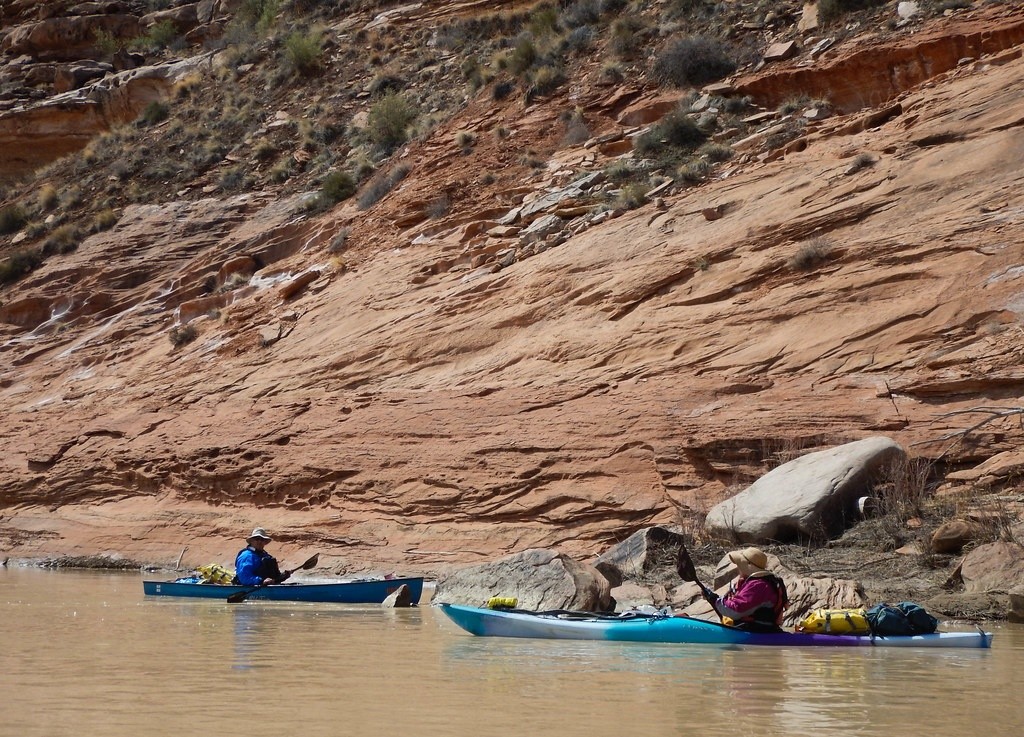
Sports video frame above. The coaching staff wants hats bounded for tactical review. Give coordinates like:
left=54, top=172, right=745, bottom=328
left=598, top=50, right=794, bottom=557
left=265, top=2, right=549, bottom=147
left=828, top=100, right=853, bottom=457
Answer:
left=246, top=528, right=272, bottom=545
left=729, top=548, right=770, bottom=577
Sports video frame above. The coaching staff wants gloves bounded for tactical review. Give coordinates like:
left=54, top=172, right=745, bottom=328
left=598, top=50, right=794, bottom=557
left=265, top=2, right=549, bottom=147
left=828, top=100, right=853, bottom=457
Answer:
left=701, top=587, right=719, bottom=604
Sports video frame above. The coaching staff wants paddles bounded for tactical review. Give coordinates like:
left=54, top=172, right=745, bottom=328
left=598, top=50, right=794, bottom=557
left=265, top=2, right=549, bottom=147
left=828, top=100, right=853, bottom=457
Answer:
left=677, top=545, right=725, bottom=623
left=227, top=552, right=319, bottom=603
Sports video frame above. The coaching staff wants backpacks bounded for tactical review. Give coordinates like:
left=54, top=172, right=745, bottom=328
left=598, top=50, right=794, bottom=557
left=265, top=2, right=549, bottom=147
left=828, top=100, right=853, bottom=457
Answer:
left=798, top=607, right=870, bottom=636
left=865, top=602, right=937, bottom=638
left=199, top=564, right=235, bottom=585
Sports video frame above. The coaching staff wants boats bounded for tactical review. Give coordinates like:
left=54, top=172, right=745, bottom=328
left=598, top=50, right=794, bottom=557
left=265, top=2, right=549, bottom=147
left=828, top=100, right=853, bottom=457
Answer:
left=438, top=602, right=993, bottom=648
left=142, top=576, right=424, bottom=606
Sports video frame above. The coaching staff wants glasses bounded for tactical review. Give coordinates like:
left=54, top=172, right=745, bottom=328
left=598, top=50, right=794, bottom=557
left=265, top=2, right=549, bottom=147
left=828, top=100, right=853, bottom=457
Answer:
left=253, top=536, right=266, bottom=542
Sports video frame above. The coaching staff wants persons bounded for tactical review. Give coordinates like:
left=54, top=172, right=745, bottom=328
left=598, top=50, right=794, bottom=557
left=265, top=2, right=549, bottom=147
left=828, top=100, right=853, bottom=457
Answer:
left=701, top=547, right=790, bottom=626
left=235, top=527, right=293, bottom=584
